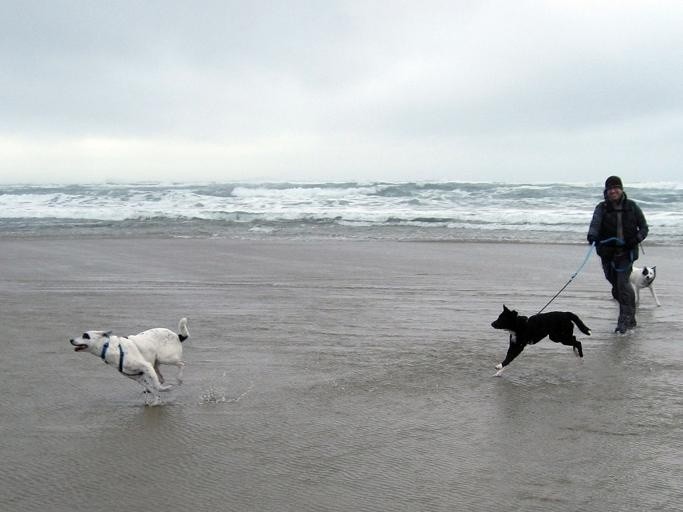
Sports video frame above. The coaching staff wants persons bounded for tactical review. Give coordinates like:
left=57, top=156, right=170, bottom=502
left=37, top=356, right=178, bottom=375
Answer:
left=586, top=174, right=648, bottom=338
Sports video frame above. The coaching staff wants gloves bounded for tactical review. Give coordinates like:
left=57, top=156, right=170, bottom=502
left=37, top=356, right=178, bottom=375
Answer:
left=588, top=235, right=599, bottom=246
left=629, top=237, right=637, bottom=250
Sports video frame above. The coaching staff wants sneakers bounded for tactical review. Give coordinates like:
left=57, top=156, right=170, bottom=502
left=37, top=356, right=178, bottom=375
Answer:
left=615, top=321, right=635, bottom=334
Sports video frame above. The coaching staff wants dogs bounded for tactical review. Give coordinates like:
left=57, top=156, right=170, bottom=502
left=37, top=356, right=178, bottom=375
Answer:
left=69, top=316, right=190, bottom=408
left=490, top=303, right=592, bottom=378
left=628, top=265, right=662, bottom=307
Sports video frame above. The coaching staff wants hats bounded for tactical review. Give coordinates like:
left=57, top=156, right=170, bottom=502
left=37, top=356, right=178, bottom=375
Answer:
left=605, top=176, right=622, bottom=190
